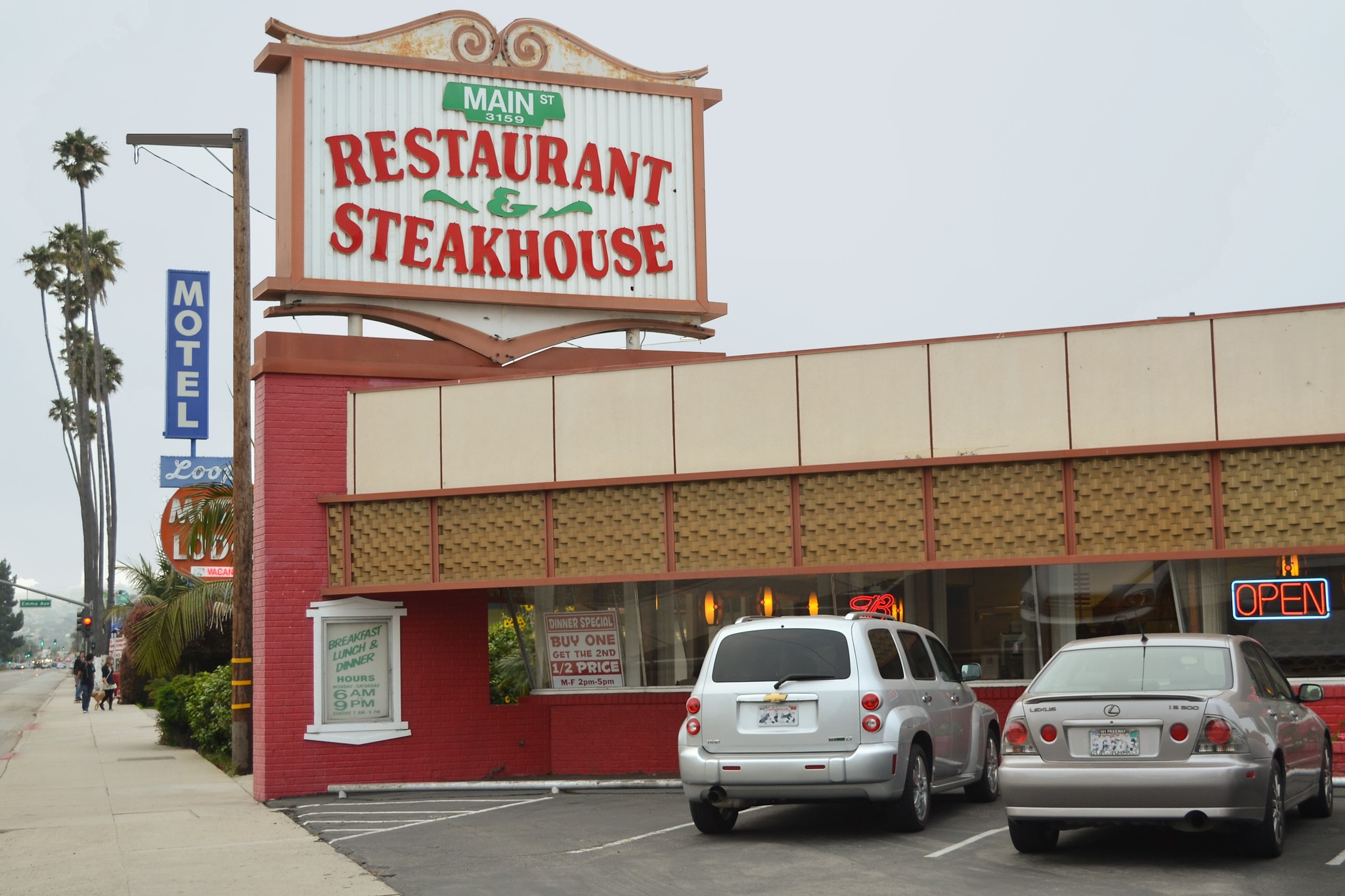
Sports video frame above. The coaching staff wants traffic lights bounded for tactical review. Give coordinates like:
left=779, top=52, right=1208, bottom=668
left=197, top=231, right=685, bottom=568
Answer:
left=63, top=647, right=65, bottom=651
left=25, top=652, right=31, bottom=658
left=76, top=609, right=94, bottom=639
left=8, top=661, right=11, bottom=666
left=53, top=639, right=57, bottom=646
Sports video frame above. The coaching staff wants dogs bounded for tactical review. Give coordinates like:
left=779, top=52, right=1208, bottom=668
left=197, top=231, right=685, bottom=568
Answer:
left=91, top=691, right=109, bottom=710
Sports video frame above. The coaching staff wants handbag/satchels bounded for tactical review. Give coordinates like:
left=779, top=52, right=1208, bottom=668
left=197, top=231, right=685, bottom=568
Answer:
left=98, top=682, right=105, bottom=696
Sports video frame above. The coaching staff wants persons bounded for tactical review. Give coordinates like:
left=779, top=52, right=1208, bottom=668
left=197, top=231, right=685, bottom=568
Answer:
left=99, top=656, right=117, bottom=711
left=73, top=651, right=86, bottom=703
left=75, top=653, right=96, bottom=713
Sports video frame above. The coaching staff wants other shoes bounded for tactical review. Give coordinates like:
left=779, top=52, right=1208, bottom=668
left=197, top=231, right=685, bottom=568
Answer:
left=74, top=697, right=82, bottom=701
left=109, top=709, right=113, bottom=711
left=83, top=708, right=88, bottom=712
left=99, top=703, right=105, bottom=710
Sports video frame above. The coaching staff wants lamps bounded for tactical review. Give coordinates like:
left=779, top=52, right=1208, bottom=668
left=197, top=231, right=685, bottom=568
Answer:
left=698, top=590, right=724, bottom=626
left=807, top=591, right=819, bottom=616
left=1276, top=555, right=1307, bottom=578
left=756, top=584, right=778, bottom=616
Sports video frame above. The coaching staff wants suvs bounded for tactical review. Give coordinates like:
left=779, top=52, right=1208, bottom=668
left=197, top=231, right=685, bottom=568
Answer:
left=678, top=612, right=1002, bottom=833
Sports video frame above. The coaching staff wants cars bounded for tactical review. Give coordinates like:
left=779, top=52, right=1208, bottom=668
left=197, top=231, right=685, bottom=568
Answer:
left=10, top=663, right=27, bottom=670
left=998, top=633, right=1333, bottom=859
left=51, top=661, right=66, bottom=669
left=57, top=657, right=72, bottom=662
left=30, top=658, right=52, bottom=669
left=70, top=663, right=74, bottom=668
